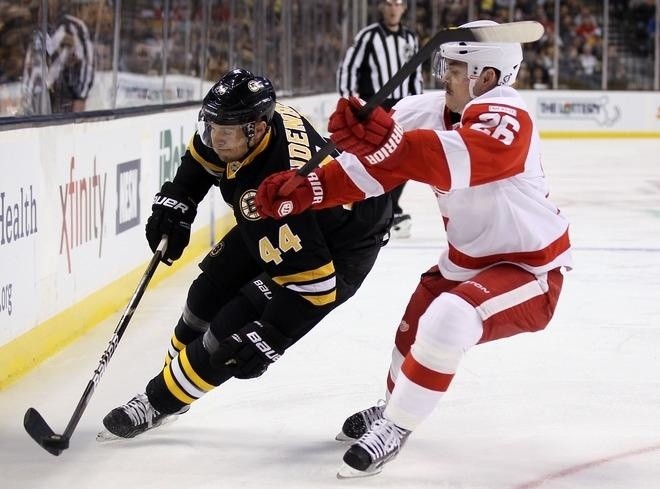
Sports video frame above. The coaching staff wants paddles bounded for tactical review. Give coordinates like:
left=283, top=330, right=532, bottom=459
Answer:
left=22, top=232, right=169, bottom=456
left=278, top=21, right=546, bottom=199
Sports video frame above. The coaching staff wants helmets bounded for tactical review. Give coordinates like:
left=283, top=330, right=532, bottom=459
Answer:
left=196, top=67, right=279, bottom=127
left=437, top=19, right=525, bottom=100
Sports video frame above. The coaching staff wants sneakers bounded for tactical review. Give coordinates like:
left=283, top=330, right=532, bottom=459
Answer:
left=342, top=417, right=414, bottom=472
left=395, top=212, right=412, bottom=227
left=103, top=392, right=168, bottom=438
left=340, top=404, right=389, bottom=438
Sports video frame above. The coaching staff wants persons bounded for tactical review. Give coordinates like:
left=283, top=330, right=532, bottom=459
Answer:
left=608, top=1, right=658, bottom=63
left=2, top=2, right=113, bottom=86
left=102, top=67, right=397, bottom=440
left=335, top=1, right=425, bottom=234
left=254, top=17, right=577, bottom=478
left=120, top=1, right=632, bottom=91
left=335, top=0, right=425, bottom=234
left=254, top=17, right=577, bottom=473
left=20, top=3, right=97, bottom=113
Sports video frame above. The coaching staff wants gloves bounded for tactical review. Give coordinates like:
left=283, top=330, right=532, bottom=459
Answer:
left=145, top=180, right=199, bottom=267
left=326, top=95, right=408, bottom=169
left=252, top=166, right=326, bottom=219
left=212, top=322, right=286, bottom=381
